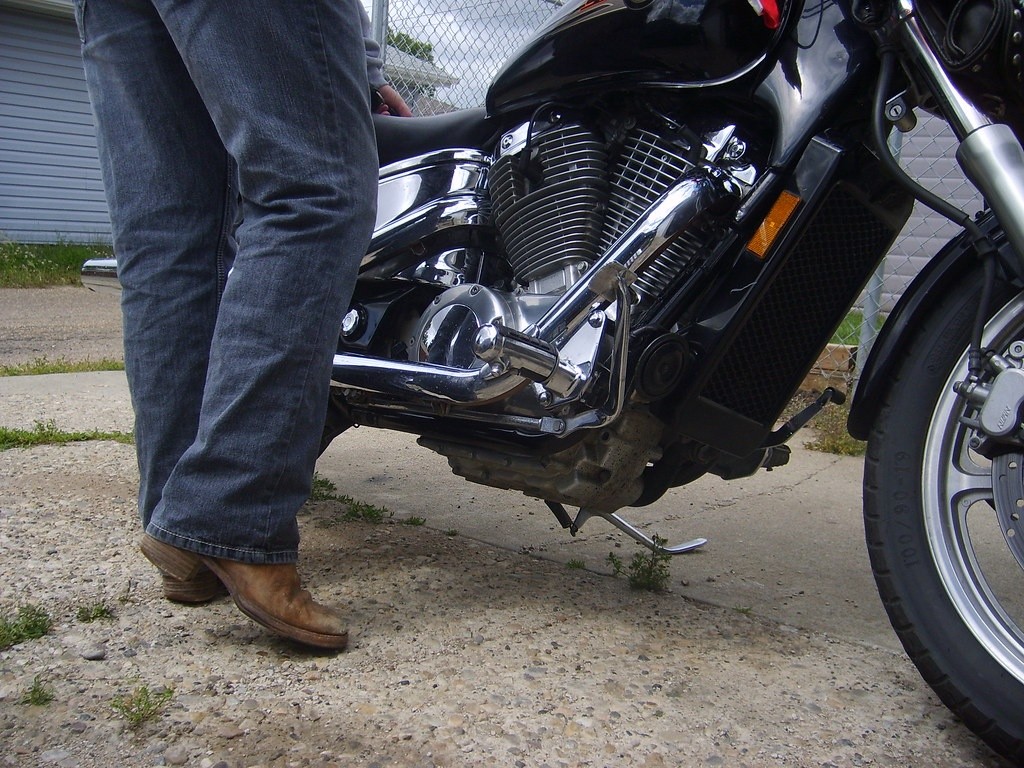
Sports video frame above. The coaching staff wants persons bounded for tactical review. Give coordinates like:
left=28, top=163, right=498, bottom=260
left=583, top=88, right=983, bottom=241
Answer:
left=72, top=0, right=412, bottom=650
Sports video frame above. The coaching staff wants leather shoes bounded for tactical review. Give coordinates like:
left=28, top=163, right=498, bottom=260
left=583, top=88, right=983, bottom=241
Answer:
left=137, top=533, right=349, bottom=650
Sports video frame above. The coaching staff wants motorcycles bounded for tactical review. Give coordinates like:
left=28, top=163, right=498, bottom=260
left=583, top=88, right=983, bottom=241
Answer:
left=79, top=0, right=1024, bottom=768
